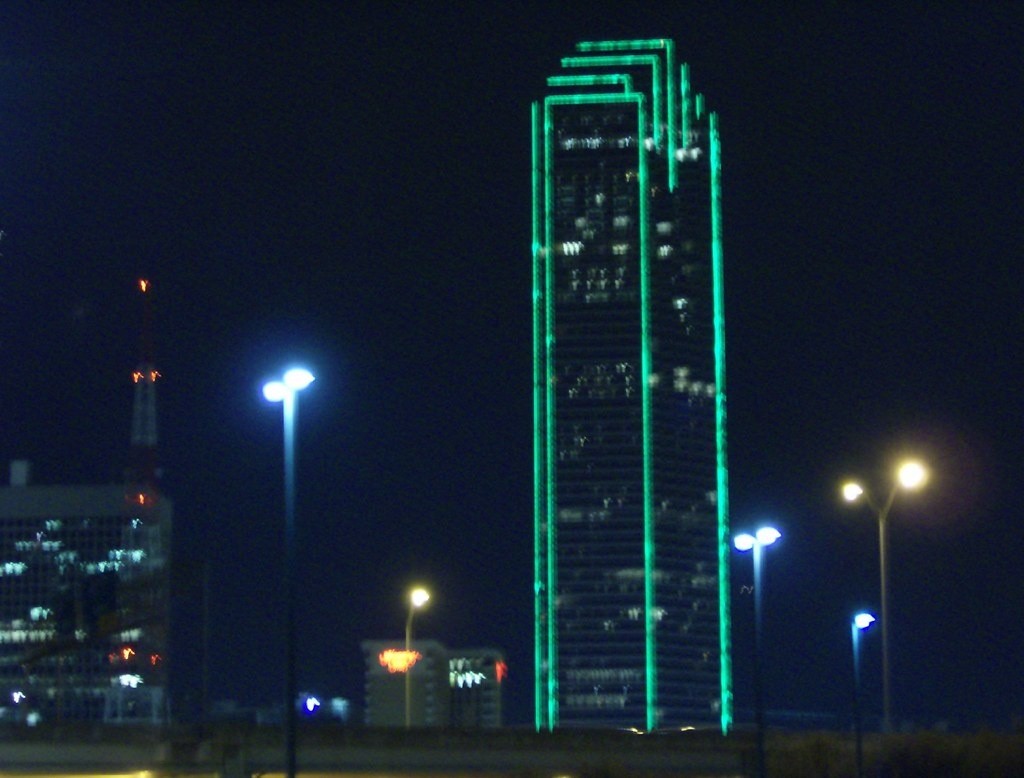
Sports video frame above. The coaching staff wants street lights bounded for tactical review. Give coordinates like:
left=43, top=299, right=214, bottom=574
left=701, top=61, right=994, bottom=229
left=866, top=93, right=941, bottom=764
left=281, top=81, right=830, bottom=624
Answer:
left=841, top=457, right=931, bottom=730
left=850, top=611, right=876, bottom=778
left=735, top=526, right=782, bottom=778
left=403, top=584, right=430, bottom=732
left=259, top=365, right=322, bottom=778
